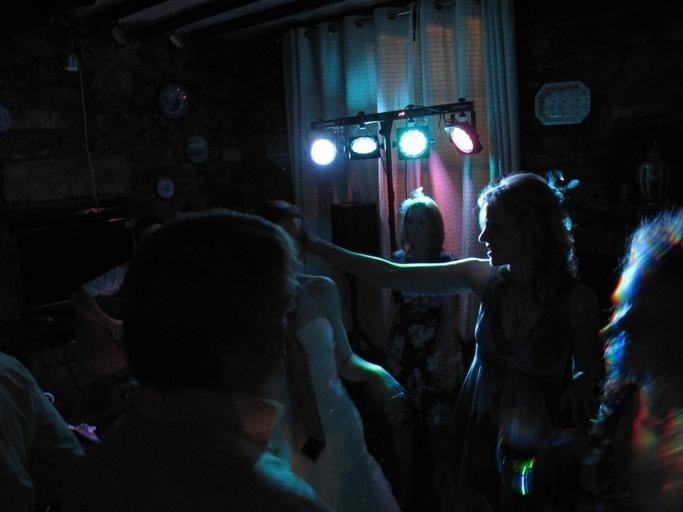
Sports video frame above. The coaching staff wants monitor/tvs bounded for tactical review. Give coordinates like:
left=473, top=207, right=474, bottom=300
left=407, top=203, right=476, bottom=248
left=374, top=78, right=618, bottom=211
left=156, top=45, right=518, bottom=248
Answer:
left=14, top=218, right=135, bottom=315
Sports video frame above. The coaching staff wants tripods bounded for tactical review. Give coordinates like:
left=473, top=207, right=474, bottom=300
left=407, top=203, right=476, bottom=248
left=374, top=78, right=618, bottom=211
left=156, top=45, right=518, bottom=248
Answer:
left=347, top=273, right=380, bottom=363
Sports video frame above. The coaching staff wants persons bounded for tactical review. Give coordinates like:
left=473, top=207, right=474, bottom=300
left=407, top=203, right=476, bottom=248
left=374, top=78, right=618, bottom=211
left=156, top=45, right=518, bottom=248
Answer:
left=0, top=170, right=409, bottom=512
left=281, top=171, right=683, bottom=511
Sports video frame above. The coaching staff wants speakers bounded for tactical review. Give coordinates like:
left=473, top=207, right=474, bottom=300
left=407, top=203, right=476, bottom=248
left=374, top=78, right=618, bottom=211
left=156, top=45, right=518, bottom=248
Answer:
left=330, top=202, right=382, bottom=258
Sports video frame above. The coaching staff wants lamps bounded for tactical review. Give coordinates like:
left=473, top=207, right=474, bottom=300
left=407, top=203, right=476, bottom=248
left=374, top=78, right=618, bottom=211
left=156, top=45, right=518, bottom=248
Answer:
left=309, top=97, right=479, bottom=168
